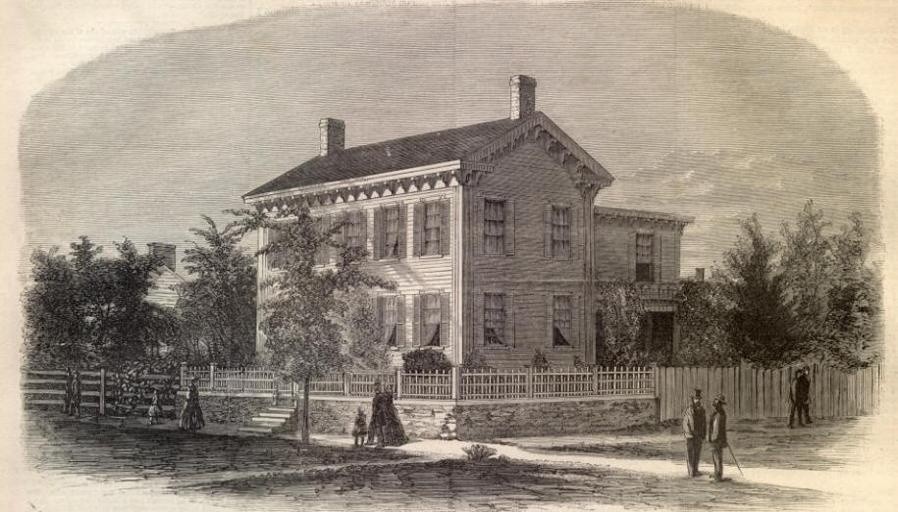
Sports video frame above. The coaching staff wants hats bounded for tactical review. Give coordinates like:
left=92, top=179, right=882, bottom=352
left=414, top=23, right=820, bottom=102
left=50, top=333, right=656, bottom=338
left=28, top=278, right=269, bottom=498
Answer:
left=690, top=389, right=701, bottom=399
left=714, top=392, right=726, bottom=404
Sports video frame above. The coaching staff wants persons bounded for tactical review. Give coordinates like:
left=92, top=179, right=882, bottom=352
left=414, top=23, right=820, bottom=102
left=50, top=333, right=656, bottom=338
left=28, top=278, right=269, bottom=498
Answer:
left=683, top=389, right=706, bottom=478
left=179, top=377, right=205, bottom=432
left=63, top=368, right=74, bottom=413
left=708, top=395, right=727, bottom=480
left=148, top=390, right=160, bottom=424
left=69, top=370, right=81, bottom=418
left=789, top=370, right=802, bottom=428
left=796, top=366, right=813, bottom=426
left=351, top=382, right=408, bottom=448
